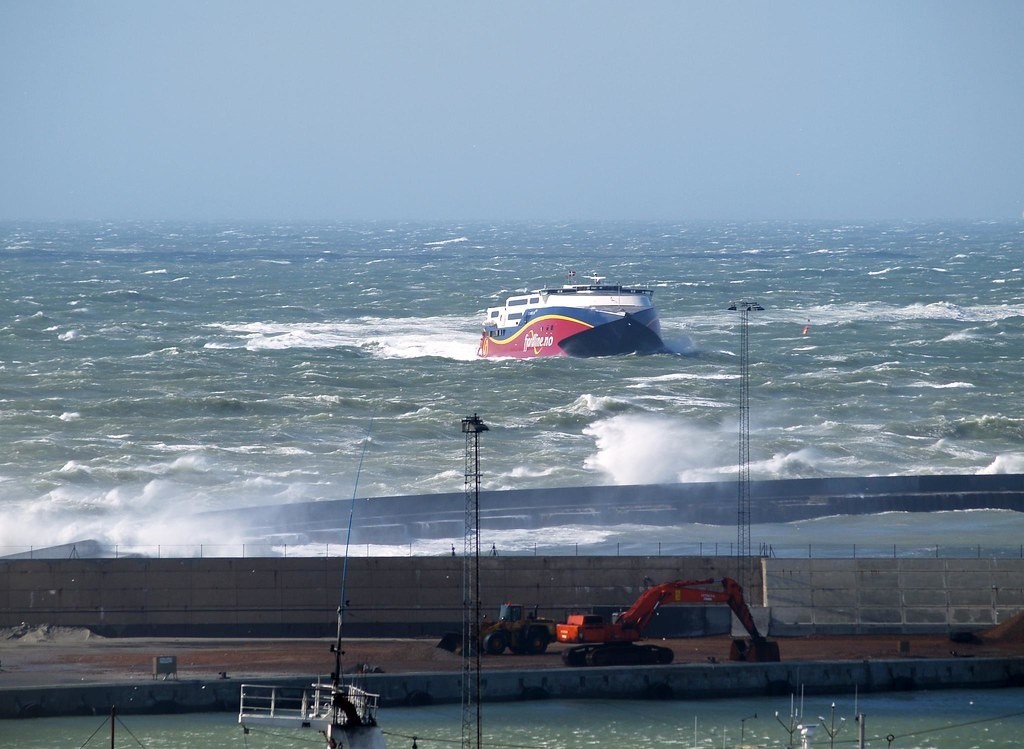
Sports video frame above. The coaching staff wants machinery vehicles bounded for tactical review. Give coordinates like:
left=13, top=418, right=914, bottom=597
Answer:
left=555, top=577, right=780, bottom=669
left=436, top=602, right=557, bottom=657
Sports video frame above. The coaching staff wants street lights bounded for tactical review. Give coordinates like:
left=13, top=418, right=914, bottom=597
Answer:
left=727, top=299, right=763, bottom=602
left=461, top=415, right=489, bottom=749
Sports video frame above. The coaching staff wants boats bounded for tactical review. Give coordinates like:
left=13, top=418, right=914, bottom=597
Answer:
left=475, top=270, right=664, bottom=360
